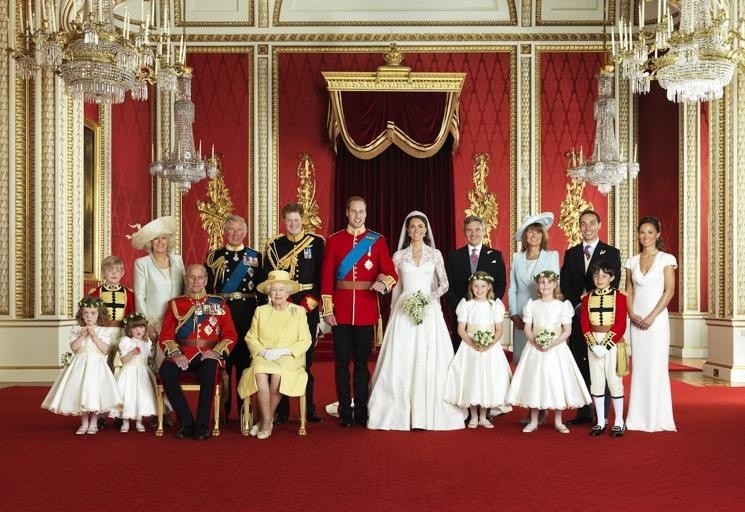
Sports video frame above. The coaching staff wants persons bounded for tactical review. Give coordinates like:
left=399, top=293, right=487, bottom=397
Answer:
left=263, top=203, right=325, bottom=424
left=88, top=256, right=135, bottom=430
left=366, top=210, right=468, bottom=431
left=444, top=270, right=513, bottom=429
left=237, top=270, right=312, bottom=439
left=560, top=210, right=622, bottom=427
left=204, top=215, right=266, bottom=423
left=40, top=294, right=125, bottom=435
left=625, top=215, right=678, bottom=432
left=444, top=216, right=506, bottom=425
left=508, top=211, right=560, bottom=425
left=580, top=259, right=628, bottom=437
left=320, top=196, right=399, bottom=427
left=159, top=264, right=238, bottom=439
left=131, top=216, right=186, bottom=428
left=505, top=271, right=592, bottom=433
left=96, top=312, right=173, bottom=433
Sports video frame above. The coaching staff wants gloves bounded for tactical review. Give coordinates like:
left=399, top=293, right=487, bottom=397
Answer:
left=596, top=345, right=606, bottom=359
left=591, top=345, right=605, bottom=358
left=264, top=350, right=291, bottom=365
left=261, top=349, right=277, bottom=360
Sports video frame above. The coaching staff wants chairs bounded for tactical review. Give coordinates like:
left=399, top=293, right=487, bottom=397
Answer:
left=155, top=358, right=226, bottom=438
left=241, top=352, right=307, bottom=437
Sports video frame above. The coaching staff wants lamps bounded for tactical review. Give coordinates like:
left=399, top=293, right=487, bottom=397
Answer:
left=15, top=0, right=187, bottom=104
left=148, top=0, right=215, bottom=191
left=610, top=1, right=745, bottom=105
left=564, top=1, right=640, bottom=197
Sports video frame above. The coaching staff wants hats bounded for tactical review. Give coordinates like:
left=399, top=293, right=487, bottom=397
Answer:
left=516, top=212, right=555, bottom=242
left=125, top=216, right=176, bottom=250
left=257, top=270, right=301, bottom=295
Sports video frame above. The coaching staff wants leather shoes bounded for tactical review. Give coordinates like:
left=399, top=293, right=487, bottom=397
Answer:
left=275, top=414, right=288, bottom=423
left=194, top=422, right=210, bottom=439
left=565, top=416, right=592, bottom=426
left=221, top=419, right=230, bottom=425
left=97, top=419, right=107, bottom=432
left=176, top=421, right=194, bottom=439
left=113, top=419, right=122, bottom=428
left=305, top=412, right=324, bottom=422
left=337, top=417, right=353, bottom=425
left=485, top=414, right=493, bottom=420
left=354, top=418, right=366, bottom=425
left=594, top=418, right=609, bottom=428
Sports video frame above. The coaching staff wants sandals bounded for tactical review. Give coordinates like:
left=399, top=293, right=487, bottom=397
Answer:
left=590, top=425, right=606, bottom=436
left=163, top=415, right=173, bottom=431
left=150, top=417, right=157, bottom=428
left=86, top=426, right=98, bottom=434
left=120, top=425, right=130, bottom=432
left=610, top=424, right=626, bottom=438
left=75, top=426, right=87, bottom=434
left=135, top=425, right=146, bottom=431
left=478, top=419, right=494, bottom=428
left=468, top=418, right=478, bottom=428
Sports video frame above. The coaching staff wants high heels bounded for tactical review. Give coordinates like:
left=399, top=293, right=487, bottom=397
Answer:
left=554, top=424, right=570, bottom=433
left=250, top=423, right=261, bottom=437
left=537, top=410, right=548, bottom=426
left=257, top=421, right=273, bottom=439
left=520, top=418, right=530, bottom=424
left=522, top=420, right=538, bottom=433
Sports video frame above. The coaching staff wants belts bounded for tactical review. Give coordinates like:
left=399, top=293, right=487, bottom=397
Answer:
left=294, top=284, right=314, bottom=290
left=98, top=321, right=124, bottom=329
left=589, top=325, right=613, bottom=331
left=218, top=292, right=256, bottom=299
left=176, top=340, right=220, bottom=348
left=334, top=281, right=375, bottom=290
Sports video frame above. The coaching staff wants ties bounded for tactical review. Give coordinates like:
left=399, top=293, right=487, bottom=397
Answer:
left=584, top=246, right=591, bottom=270
left=470, top=249, right=478, bottom=275
